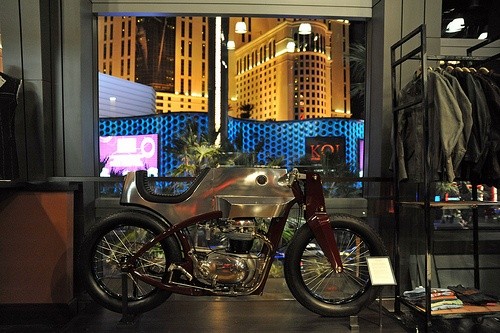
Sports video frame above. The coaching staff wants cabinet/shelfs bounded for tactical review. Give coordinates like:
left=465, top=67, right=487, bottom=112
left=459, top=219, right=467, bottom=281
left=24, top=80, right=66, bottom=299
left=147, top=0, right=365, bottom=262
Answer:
left=389, top=25, right=500, bottom=333
left=0, top=181, right=84, bottom=324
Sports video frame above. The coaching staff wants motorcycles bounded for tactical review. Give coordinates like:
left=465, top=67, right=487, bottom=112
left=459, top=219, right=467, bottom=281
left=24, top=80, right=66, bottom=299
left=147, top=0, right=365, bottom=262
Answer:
left=77, top=167, right=389, bottom=317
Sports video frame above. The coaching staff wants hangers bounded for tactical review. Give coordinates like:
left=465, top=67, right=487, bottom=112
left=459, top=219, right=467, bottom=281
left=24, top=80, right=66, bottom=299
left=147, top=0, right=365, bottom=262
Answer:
left=416, top=54, right=490, bottom=77
